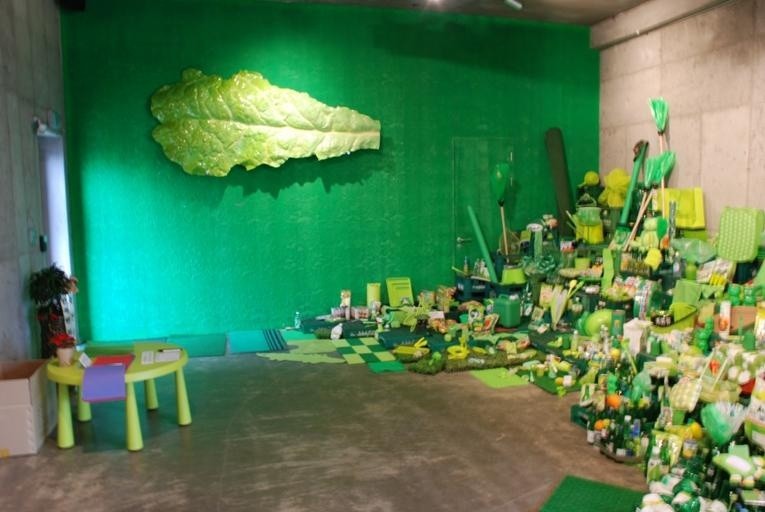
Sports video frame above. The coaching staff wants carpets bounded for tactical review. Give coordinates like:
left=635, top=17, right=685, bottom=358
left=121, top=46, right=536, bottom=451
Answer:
left=333, top=337, right=396, bottom=366
left=164, top=328, right=288, bottom=358
left=468, top=362, right=599, bottom=395
left=540, top=475, right=644, bottom=512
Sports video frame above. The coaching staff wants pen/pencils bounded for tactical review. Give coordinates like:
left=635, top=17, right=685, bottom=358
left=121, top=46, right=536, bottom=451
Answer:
left=159, top=349, right=183, bottom=352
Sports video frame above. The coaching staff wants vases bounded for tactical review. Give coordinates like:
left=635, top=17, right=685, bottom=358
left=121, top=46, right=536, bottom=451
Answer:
left=56, top=347, right=73, bottom=367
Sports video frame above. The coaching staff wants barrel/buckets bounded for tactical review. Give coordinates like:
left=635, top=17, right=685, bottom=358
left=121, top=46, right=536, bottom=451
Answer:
left=485, top=294, right=521, bottom=328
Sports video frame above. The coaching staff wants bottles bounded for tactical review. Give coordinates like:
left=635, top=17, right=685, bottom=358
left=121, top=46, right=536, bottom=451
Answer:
left=640, top=466, right=703, bottom=511
left=571, top=329, right=578, bottom=352
left=640, top=434, right=669, bottom=485
left=702, top=450, right=733, bottom=502
left=607, top=402, right=647, bottom=454
left=463, top=256, right=469, bottom=276
left=672, top=250, right=681, bottom=280
left=523, top=291, right=534, bottom=321
left=600, top=324, right=609, bottom=354
left=719, top=301, right=732, bottom=339
left=294, top=312, right=301, bottom=328
left=586, top=405, right=595, bottom=443
left=582, top=384, right=590, bottom=402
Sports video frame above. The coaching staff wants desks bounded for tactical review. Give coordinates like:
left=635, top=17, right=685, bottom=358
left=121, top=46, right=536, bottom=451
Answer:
left=47, top=341, right=193, bottom=452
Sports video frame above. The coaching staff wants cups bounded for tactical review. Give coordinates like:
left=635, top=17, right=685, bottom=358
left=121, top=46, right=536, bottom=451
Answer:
left=536, top=363, right=546, bottom=377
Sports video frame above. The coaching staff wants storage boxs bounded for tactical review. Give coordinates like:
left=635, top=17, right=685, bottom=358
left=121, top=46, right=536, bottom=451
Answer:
left=0, top=359, right=59, bottom=458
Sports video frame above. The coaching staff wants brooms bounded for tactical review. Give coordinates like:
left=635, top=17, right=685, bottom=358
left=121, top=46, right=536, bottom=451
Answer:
left=624, top=151, right=676, bottom=252
left=626, top=153, right=665, bottom=252
left=649, top=95, right=669, bottom=221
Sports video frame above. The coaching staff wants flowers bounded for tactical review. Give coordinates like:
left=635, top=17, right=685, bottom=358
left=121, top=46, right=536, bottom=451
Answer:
left=54, top=334, right=75, bottom=348
